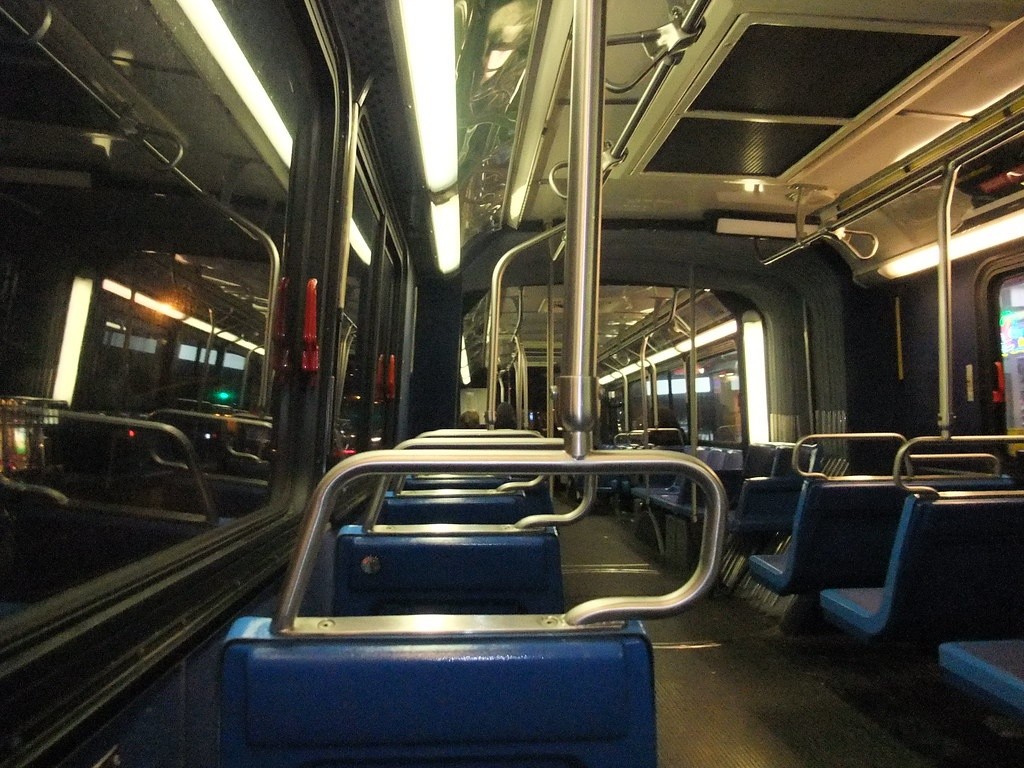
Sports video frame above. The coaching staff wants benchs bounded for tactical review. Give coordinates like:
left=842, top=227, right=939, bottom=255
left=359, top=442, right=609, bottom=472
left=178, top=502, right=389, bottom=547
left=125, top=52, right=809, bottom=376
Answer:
left=0, top=403, right=275, bottom=605
left=575, top=429, right=1024, bottom=768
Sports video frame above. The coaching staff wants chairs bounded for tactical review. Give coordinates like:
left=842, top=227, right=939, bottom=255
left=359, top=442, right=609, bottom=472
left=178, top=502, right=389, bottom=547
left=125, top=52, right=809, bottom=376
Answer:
left=218, top=428, right=729, bottom=768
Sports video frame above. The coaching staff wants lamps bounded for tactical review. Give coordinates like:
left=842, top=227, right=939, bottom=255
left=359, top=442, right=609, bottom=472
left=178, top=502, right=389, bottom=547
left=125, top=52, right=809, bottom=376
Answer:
left=598, top=318, right=738, bottom=387
left=387, top=0, right=464, bottom=281
left=877, top=198, right=1024, bottom=281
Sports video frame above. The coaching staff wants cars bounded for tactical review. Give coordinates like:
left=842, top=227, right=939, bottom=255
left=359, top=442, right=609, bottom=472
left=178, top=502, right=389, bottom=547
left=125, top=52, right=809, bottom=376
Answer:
left=0, top=394, right=382, bottom=515
